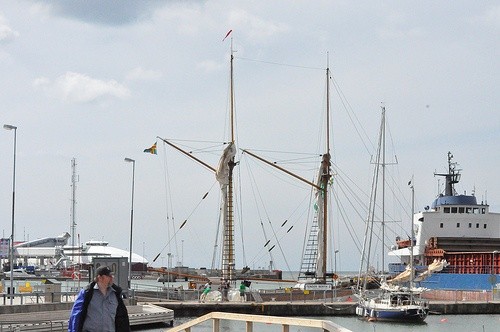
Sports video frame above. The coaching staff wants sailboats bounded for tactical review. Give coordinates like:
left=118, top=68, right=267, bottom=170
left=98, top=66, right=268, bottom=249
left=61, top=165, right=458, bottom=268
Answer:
left=356, top=107, right=431, bottom=322
left=154, top=28, right=383, bottom=313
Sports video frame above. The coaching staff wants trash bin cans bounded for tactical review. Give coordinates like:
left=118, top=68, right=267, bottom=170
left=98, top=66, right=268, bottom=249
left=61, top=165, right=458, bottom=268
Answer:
left=7, top=287, right=15, bottom=299
left=45, top=279, right=62, bottom=303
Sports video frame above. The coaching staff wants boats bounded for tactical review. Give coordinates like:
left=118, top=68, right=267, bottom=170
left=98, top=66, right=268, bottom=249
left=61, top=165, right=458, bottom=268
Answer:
left=1, top=232, right=149, bottom=271
left=386, top=151, right=500, bottom=313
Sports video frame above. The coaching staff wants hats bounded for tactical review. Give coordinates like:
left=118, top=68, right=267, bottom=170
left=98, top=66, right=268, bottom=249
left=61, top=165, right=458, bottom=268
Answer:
left=95, top=265, right=115, bottom=278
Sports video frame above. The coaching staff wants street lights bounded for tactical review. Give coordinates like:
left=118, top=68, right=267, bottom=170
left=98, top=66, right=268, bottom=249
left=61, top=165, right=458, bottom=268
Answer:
left=3, top=123, right=17, bottom=305
left=124, top=157, right=136, bottom=291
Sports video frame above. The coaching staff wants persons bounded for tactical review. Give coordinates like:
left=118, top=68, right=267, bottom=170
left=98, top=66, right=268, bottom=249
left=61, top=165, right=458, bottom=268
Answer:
left=200, top=282, right=211, bottom=303
left=68, top=265, right=131, bottom=332
left=239, top=281, right=245, bottom=302
left=216, top=286, right=223, bottom=302
